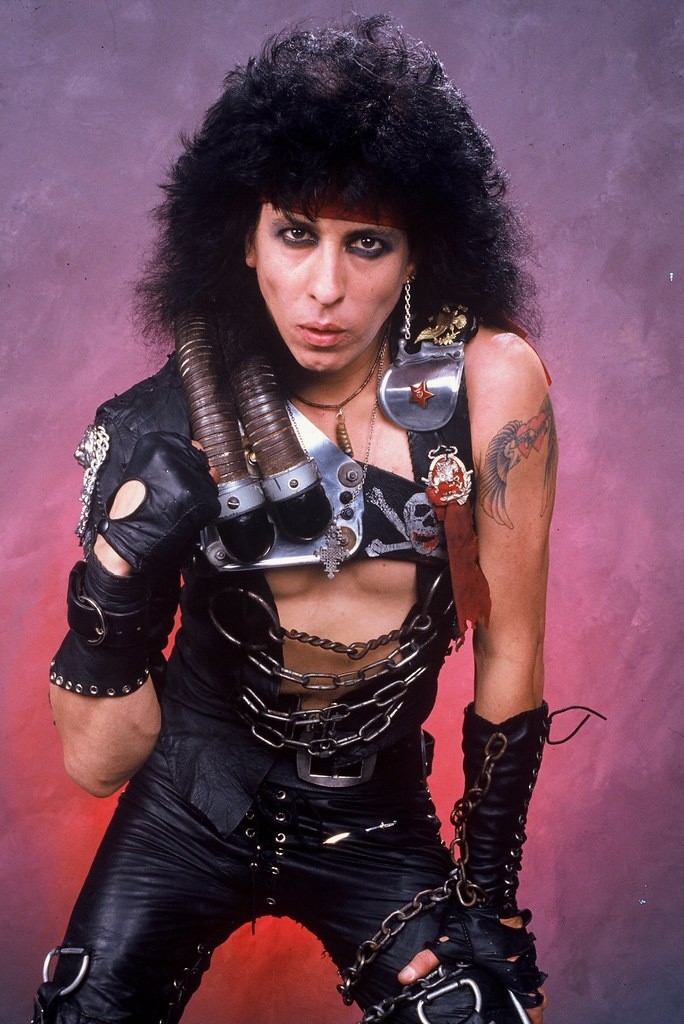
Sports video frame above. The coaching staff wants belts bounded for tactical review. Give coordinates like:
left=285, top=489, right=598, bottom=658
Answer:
left=263, top=729, right=435, bottom=789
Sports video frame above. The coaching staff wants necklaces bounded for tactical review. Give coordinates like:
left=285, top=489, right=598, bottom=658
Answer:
left=286, top=326, right=386, bottom=579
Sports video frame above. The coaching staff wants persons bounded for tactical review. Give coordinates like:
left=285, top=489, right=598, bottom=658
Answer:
left=1, top=16, right=557, bottom=1024
